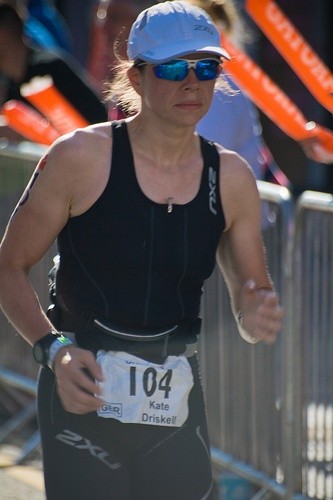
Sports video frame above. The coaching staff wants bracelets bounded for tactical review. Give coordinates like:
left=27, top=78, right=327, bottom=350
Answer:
left=48, top=336, right=74, bottom=374
left=238, top=286, right=276, bottom=322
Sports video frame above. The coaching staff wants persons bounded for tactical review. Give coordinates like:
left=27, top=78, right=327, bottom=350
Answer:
left=0, top=0, right=285, bottom=500
left=0, top=0, right=278, bottom=272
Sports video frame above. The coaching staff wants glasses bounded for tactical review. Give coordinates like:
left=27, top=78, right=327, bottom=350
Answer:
left=136, top=58, right=220, bottom=81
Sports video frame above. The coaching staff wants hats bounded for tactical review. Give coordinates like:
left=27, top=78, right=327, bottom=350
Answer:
left=127, top=1, right=230, bottom=63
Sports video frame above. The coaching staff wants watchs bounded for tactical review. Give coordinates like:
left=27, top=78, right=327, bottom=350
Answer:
left=32, top=331, right=66, bottom=369
